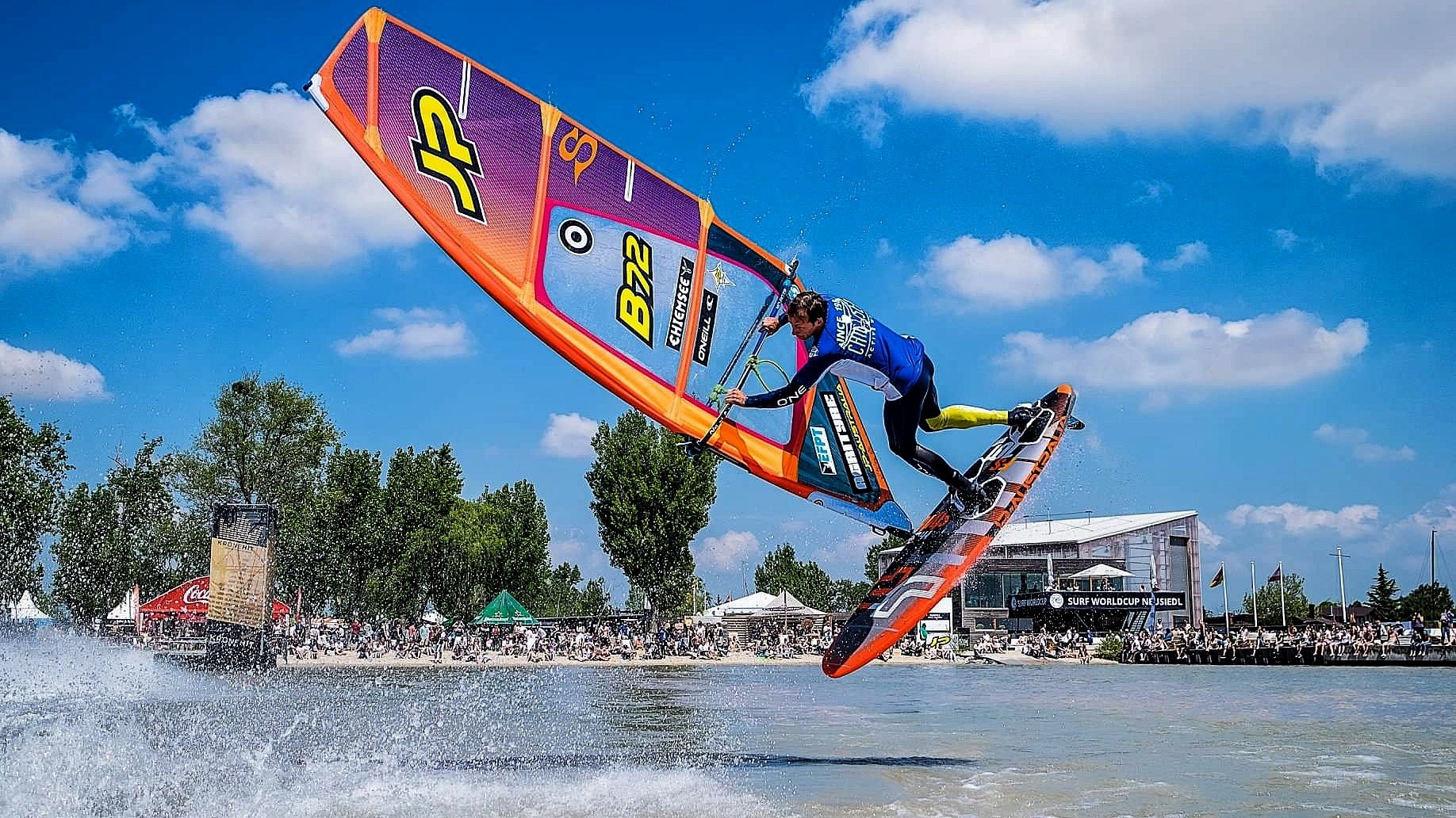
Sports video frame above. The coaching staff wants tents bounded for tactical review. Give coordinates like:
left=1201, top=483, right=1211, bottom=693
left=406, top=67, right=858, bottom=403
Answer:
left=703, top=590, right=827, bottom=617
left=139, top=575, right=291, bottom=638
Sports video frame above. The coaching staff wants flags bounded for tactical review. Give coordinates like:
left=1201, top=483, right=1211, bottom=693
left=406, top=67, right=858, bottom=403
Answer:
left=1268, top=565, right=1281, bottom=582
left=1210, top=566, right=1223, bottom=588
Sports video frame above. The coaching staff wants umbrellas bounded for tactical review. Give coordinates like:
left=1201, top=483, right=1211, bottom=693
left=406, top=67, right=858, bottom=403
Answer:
left=468, top=589, right=540, bottom=624
left=1047, top=553, right=1054, bottom=585
left=1149, top=554, right=1158, bottom=591
left=1068, top=563, right=1136, bottom=583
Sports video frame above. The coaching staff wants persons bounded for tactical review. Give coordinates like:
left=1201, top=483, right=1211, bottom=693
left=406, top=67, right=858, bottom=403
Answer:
left=0, top=615, right=848, bottom=663
left=875, top=604, right=1456, bottom=665
left=725, top=291, right=1034, bottom=500
left=1009, top=576, right=1157, bottom=594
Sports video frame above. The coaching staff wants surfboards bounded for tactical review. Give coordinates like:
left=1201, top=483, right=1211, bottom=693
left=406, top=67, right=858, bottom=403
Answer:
left=822, top=383, right=1086, bottom=679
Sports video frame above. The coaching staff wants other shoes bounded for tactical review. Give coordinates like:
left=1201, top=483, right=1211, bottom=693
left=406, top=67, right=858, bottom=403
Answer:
left=948, top=484, right=986, bottom=507
left=1008, top=402, right=1033, bottom=425
left=1232, top=656, right=1236, bottom=661
left=1220, top=655, right=1226, bottom=659
